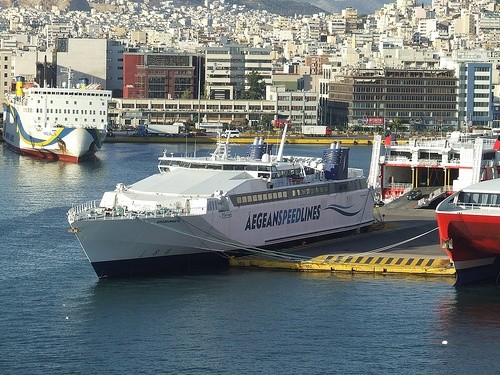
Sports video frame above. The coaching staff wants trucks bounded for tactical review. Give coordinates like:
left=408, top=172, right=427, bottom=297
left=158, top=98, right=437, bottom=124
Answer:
left=65, top=120, right=387, bottom=280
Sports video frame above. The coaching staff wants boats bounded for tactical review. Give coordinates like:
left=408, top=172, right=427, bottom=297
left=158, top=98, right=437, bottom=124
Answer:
left=435, top=176, right=500, bottom=290
left=1, top=54, right=113, bottom=164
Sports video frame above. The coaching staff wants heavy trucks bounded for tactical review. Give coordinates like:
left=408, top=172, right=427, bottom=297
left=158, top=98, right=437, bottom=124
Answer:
left=302, top=125, right=332, bottom=137
left=135, top=124, right=179, bottom=137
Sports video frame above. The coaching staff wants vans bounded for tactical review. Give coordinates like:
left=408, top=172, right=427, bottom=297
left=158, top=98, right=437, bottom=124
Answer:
left=221, top=129, right=240, bottom=138
